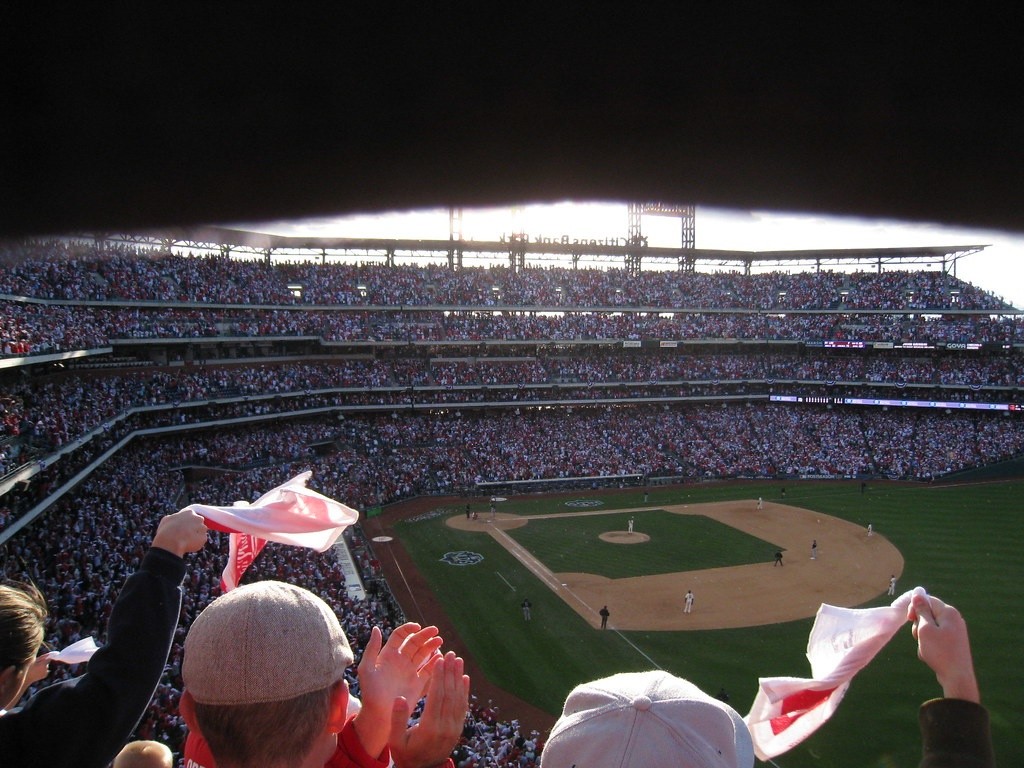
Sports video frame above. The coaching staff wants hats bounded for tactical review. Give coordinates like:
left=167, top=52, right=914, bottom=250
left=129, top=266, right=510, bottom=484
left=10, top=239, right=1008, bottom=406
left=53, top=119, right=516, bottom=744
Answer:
left=182, top=580, right=354, bottom=705
left=540, top=670, right=754, bottom=768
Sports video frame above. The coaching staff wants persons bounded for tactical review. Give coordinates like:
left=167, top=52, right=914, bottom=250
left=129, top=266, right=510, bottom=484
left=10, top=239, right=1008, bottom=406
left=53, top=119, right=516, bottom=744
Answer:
left=598, top=606, right=609, bottom=630
left=0, top=238, right=1024, bottom=768
left=683, top=590, right=695, bottom=615
left=522, top=598, right=532, bottom=622
